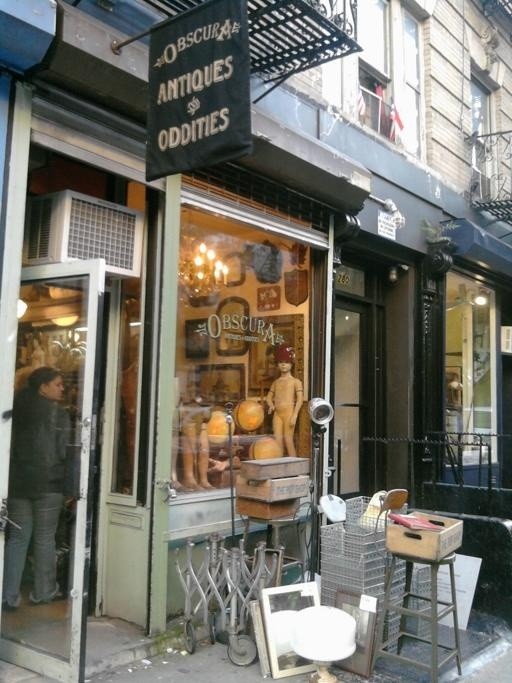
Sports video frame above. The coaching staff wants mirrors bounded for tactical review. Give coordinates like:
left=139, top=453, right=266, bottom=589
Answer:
left=258, top=579, right=325, bottom=678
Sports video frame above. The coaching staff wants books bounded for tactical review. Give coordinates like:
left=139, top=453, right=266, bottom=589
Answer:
left=388, top=511, right=444, bottom=532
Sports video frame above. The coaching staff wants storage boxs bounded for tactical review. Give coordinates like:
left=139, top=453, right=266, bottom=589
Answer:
left=235, top=455, right=312, bottom=519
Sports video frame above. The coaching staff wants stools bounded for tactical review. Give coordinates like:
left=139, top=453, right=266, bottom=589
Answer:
left=368, top=552, right=462, bottom=682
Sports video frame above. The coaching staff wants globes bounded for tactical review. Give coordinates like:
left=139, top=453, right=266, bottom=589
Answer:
left=206, top=399, right=280, bottom=460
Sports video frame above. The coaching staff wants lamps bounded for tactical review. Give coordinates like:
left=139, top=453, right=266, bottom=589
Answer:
left=306, top=397, right=335, bottom=581
left=179, top=207, right=228, bottom=308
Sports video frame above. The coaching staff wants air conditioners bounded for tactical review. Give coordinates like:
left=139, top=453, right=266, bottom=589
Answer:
left=23, top=189, right=147, bottom=281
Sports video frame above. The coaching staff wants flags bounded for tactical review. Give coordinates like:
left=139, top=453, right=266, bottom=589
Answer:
left=387, top=96, right=405, bottom=144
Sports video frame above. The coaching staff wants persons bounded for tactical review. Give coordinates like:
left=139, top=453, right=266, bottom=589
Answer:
left=2, top=363, right=81, bottom=610
left=267, top=342, right=305, bottom=456
left=178, top=365, right=217, bottom=492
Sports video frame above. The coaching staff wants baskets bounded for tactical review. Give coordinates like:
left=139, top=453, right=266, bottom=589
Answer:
left=320, top=496, right=432, bottom=658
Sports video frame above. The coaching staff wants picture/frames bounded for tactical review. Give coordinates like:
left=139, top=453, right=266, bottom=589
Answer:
left=185, top=251, right=305, bottom=410
